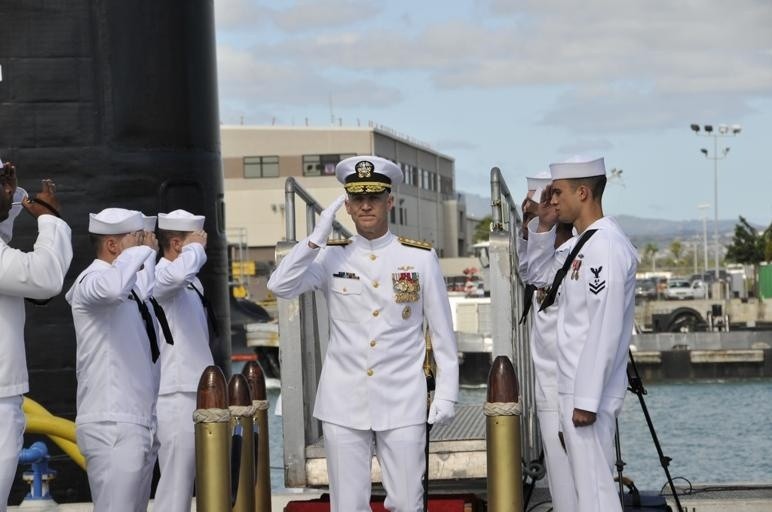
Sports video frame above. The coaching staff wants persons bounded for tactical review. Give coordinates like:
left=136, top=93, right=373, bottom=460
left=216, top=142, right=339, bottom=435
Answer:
left=517, top=154, right=637, bottom=512
left=266, top=155, right=460, bottom=512
left=0, top=159, right=75, bottom=512
left=65, top=209, right=214, bottom=512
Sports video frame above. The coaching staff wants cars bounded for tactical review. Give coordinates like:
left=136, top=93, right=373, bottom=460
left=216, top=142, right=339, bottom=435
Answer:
left=635, top=261, right=747, bottom=300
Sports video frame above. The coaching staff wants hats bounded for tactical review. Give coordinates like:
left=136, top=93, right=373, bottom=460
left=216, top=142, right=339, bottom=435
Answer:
left=157, top=209, right=206, bottom=233
left=548, top=157, right=608, bottom=179
left=142, top=211, right=159, bottom=233
left=334, top=156, right=405, bottom=196
left=87, top=206, right=143, bottom=235
left=523, top=171, right=553, bottom=191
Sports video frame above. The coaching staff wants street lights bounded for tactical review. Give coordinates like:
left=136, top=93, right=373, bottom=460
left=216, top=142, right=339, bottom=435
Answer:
left=696, top=201, right=715, bottom=273
left=606, top=164, right=628, bottom=190
left=689, top=118, right=745, bottom=275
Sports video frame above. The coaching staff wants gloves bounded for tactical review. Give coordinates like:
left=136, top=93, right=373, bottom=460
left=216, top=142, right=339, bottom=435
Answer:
left=308, top=191, right=347, bottom=250
left=428, top=398, right=457, bottom=425
